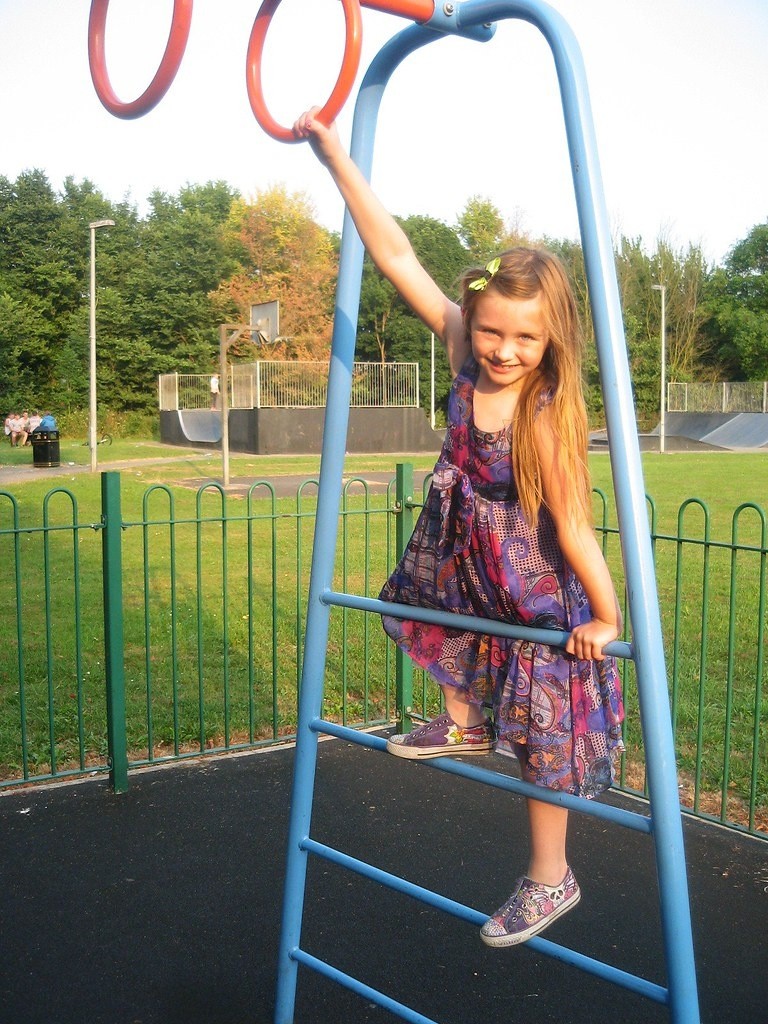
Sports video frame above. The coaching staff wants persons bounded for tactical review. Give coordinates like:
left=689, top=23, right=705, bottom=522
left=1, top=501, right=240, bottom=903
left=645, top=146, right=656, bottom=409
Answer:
left=292, top=105, right=626, bottom=947
left=4, top=410, right=56, bottom=448
left=209, top=372, right=221, bottom=411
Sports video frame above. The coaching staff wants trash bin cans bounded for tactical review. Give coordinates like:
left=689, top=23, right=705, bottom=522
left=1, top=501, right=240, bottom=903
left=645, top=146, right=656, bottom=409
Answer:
left=32, top=426, right=60, bottom=467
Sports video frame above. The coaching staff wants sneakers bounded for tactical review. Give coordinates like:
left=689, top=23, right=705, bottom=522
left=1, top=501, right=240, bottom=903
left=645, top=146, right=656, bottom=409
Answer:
left=479, top=863, right=581, bottom=947
left=387, top=712, right=498, bottom=759
